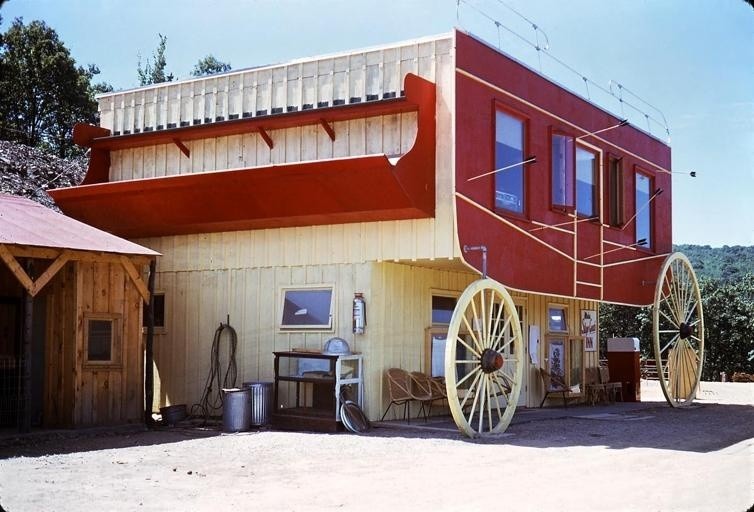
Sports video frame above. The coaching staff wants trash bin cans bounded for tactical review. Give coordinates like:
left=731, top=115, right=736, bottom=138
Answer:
left=221, top=382, right=274, bottom=433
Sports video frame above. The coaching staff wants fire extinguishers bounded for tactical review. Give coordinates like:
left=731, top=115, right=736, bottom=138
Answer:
left=352, top=293, right=366, bottom=334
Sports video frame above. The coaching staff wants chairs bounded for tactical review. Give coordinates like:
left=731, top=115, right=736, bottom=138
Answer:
left=379, top=367, right=456, bottom=426
left=539, top=367, right=573, bottom=411
left=578, top=365, right=624, bottom=408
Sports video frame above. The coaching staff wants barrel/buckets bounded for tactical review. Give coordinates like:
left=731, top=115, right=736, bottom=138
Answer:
left=224, top=390, right=251, bottom=433
left=244, top=382, right=273, bottom=426
